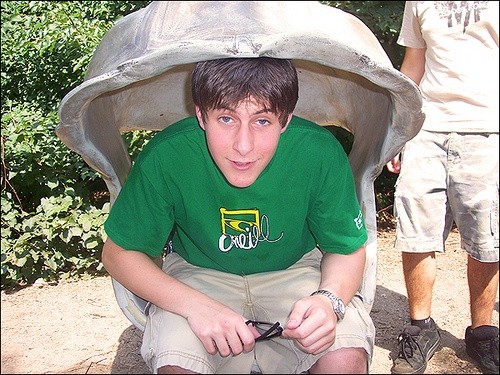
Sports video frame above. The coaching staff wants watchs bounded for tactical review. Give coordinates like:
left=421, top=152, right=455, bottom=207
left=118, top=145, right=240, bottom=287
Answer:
left=311, top=290, right=346, bottom=322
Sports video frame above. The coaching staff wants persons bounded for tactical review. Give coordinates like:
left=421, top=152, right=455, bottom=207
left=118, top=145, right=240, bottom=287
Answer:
left=99, top=56, right=377, bottom=375
left=385, top=0, right=500, bottom=374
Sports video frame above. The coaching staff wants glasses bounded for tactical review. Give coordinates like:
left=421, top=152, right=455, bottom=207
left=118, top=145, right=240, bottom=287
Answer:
left=241, top=315, right=286, bottom=345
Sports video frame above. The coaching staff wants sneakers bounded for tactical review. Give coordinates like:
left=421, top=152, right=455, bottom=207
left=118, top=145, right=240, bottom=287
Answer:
left=392, top=320, right=441, bottom=373
left=465, top=324, right=500, bottom=375
